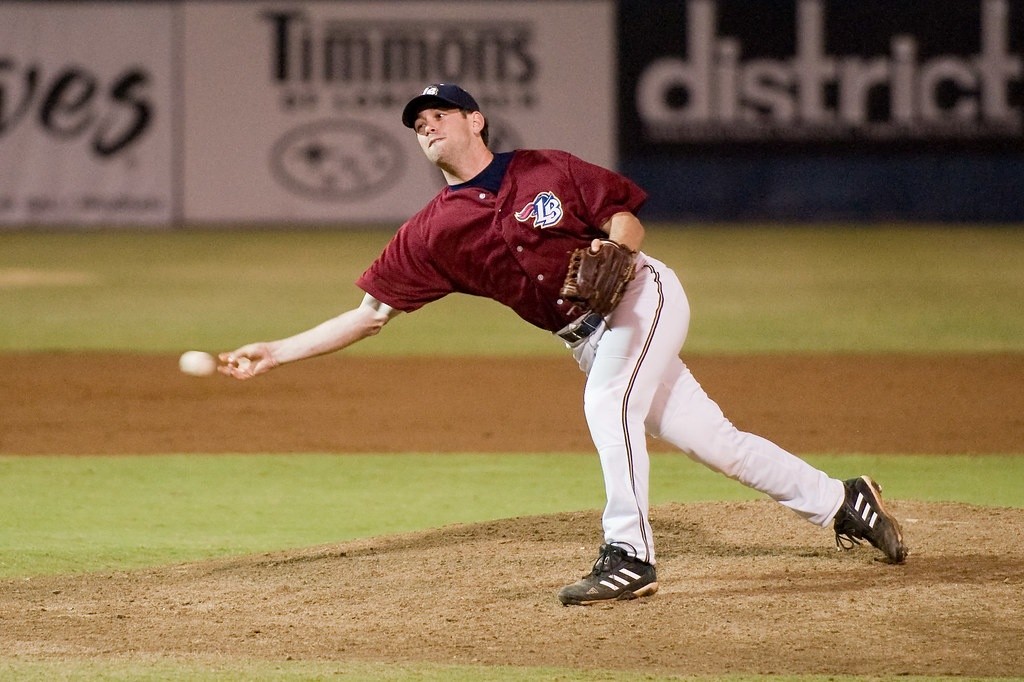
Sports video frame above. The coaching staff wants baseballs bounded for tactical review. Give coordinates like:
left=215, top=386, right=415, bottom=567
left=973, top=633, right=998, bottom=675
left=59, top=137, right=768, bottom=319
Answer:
left=178, top=348, right=220, bottom=378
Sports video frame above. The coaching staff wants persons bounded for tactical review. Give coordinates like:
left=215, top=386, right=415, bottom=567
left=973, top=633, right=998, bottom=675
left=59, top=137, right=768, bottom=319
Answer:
left=217, top=82, right=908, bottom=605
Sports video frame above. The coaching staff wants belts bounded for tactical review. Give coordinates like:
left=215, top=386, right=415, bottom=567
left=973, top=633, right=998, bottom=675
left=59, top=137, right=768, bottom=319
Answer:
left=559, top=314, right=602, bottom=343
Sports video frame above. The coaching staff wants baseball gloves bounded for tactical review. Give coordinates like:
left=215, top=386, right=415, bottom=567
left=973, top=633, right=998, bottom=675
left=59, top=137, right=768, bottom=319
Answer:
left=559, top=238, right=636, bottom=317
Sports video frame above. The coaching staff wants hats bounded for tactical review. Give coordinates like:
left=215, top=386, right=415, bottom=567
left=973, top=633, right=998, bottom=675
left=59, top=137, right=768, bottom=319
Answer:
left=402, top=84, right=479, bottom=128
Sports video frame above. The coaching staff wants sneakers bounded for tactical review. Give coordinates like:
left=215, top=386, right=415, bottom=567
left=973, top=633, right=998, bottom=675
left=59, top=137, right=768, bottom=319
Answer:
left=558, top=541, right=658, bottom=605
left=833, top=475, right=904, bottom=563
